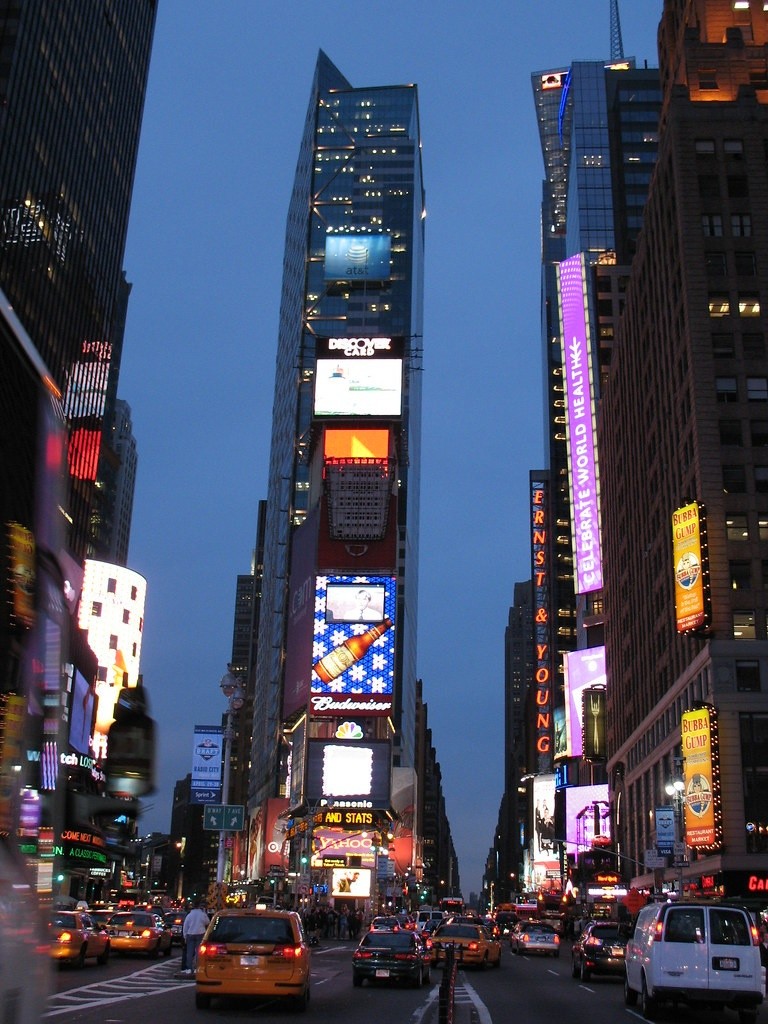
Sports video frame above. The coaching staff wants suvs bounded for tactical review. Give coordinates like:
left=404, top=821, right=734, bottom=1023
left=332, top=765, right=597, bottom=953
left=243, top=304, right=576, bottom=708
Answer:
left=569, top=919, right=635, bottom=985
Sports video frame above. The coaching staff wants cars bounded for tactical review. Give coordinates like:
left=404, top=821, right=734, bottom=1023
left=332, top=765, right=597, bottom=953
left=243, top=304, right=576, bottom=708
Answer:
left=102, top=891, right=210, bottom=947
left=424, top=922, right=502, bottom=969
left=43, top=910, right=115, bottom=969
left=365, top=896, right=576, bottom=954
left=99, top=912, right=174, bottom=957
left=352, top=929, right=432, bottom=990
left=510, top=920, right=560, bottom=956
left=194, top=906, right=319, bottom=999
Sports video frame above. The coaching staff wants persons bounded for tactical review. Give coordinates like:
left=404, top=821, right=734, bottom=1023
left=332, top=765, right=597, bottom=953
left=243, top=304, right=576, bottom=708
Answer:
left=183, top=900, right=210, bottom=973
left=297, top=904, right=365, bottom=941
left=182, top=901, right=194, bottom=974
left=760, top=920, right=768, bottom=995
left=55, top=900, right=77, bottom=911
left=422, top=916, right=435, bottom=932
left=563, top=914, right=619, bottom=940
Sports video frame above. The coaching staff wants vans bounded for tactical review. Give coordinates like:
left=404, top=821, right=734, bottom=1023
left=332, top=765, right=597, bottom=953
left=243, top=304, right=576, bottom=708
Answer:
left=625, top=901, right=763, bottom=1020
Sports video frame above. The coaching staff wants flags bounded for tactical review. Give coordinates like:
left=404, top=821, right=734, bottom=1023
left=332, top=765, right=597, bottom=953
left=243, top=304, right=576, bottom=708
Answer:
left=378, top=855, right=389, bottom=884
left=388, top=859, right=395, bottom=882
left=408, top=874, right=416, bottom=892
